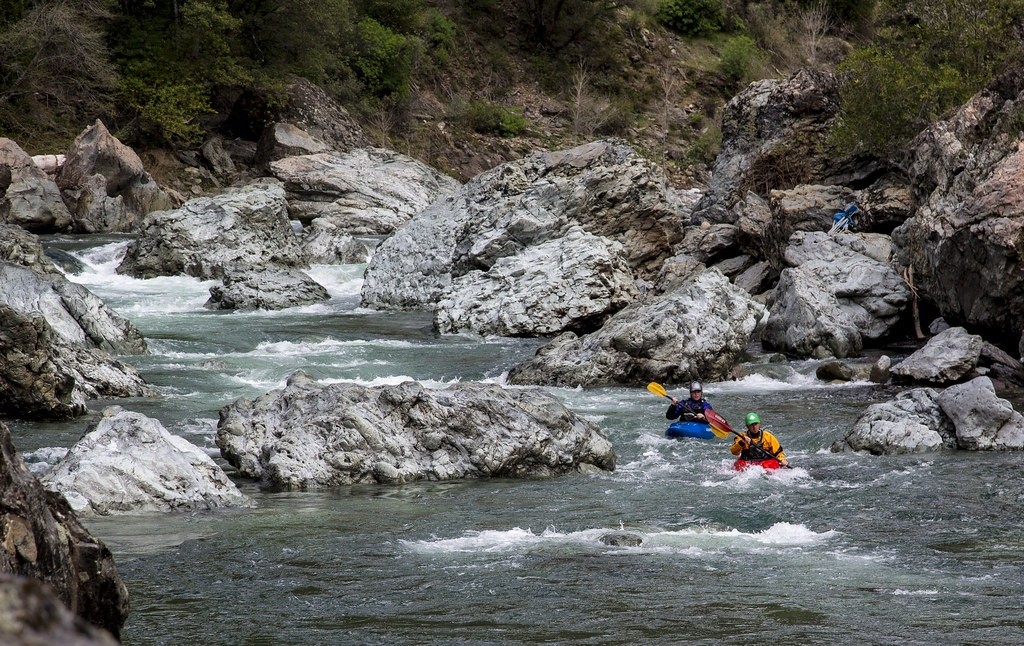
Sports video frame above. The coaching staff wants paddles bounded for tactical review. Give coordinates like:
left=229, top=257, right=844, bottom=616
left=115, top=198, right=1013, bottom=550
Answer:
left=647, top=382, right=730, bottom=439
left=705, top=408, right=793, bottom=469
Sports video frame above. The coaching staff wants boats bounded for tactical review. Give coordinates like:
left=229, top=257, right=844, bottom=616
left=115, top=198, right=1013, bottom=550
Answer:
left=668, top=420, right=715, bottom=438
left=735, top=459, right=781, bottom=470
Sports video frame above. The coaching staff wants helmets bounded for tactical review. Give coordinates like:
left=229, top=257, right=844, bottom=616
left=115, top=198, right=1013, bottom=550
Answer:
left=745, top=413, right=761, bottom=425
left=690, top=381, right=702, bottom=392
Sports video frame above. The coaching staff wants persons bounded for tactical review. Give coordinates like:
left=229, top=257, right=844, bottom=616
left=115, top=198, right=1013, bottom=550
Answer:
left=730, top=413, right=788, bottom=467
left=666, top=382, right=713, bottom=424
